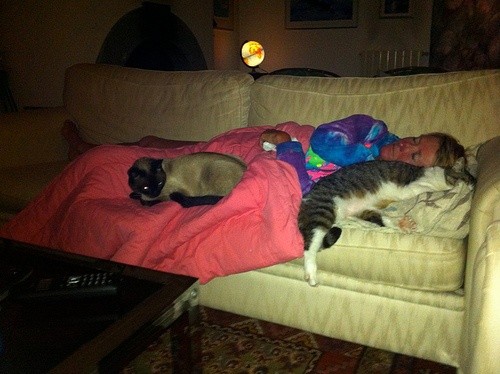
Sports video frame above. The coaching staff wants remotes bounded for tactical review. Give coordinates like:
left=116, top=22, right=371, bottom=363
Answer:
left=29, top=270, right=119, bottom=297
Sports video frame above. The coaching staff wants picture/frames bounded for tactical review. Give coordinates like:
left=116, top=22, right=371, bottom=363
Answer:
left=284, top=0, right=359, bottom=30
left=379, top=0, right=416, bottom=20
left=212, top=0, right=234, bottom=31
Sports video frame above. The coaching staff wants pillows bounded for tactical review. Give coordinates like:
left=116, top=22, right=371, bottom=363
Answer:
left=379, top=143, right=482, bottom=237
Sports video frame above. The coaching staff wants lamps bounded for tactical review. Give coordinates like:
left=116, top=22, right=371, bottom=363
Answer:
left=240, top=40, right=265, bottom=73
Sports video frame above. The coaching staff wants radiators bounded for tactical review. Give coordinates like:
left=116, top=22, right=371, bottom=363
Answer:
left=358, top=50, right=428, bottom=70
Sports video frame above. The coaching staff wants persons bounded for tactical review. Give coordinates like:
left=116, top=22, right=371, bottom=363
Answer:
left=61, top=114, right=464, bottom=197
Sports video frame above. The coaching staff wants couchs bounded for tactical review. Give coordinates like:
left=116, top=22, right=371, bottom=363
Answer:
left=0, top=63, right=500, bottom=374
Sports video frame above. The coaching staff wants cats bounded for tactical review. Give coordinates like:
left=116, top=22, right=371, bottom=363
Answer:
left=127, top=152, right=247, bottom=208
left=297, top=157, right=477, bottom=287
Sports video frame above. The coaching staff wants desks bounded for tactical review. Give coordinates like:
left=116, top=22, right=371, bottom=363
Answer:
left=0, top=236, right=203, bottom=374
